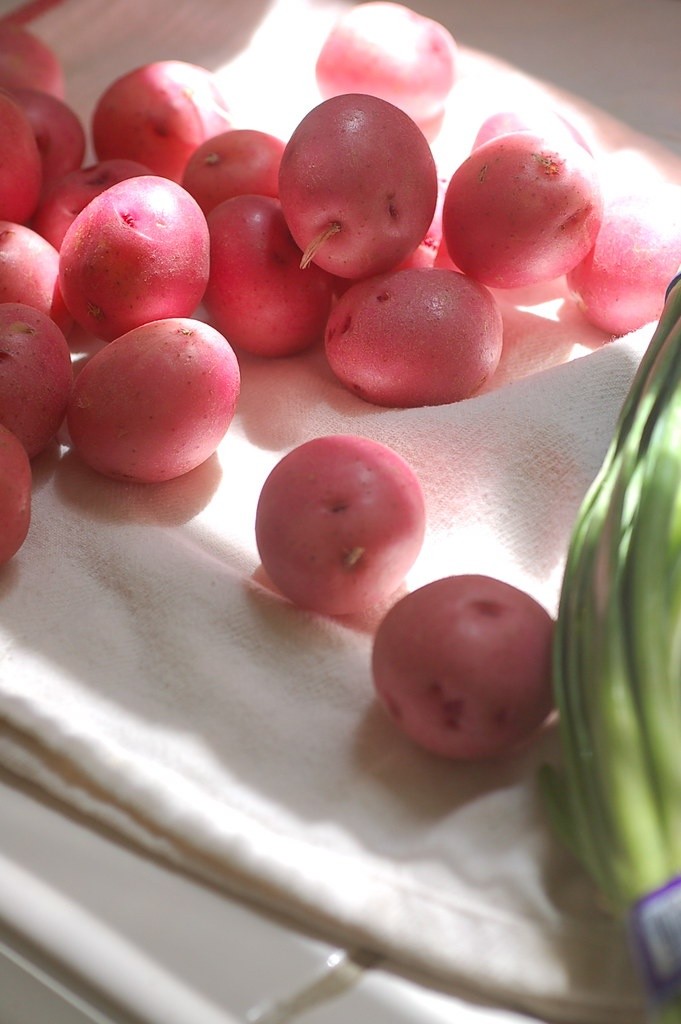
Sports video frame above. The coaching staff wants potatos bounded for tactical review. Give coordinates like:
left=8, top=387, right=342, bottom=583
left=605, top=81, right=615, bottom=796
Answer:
left=0, top=0, right=681, bottom=766
